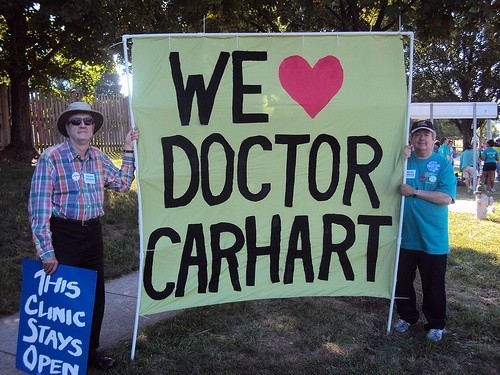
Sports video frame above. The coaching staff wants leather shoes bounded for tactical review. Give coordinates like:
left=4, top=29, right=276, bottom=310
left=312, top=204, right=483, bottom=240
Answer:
left=90, top=356, right=118, bottom=370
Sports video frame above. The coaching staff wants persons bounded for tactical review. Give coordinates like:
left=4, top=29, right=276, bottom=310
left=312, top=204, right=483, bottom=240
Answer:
left=480, top=137, right=500, bottom=193
left=460, top=142, right=479, bottom=192
left=393, top=119, right=457, bottom=340
left=438, top=137, right=451, bottom=162
left=28, top=102, right=140, bottom=370
left=448, top=140, right=456, bottom=166
left=433, top=140, right=440, bottom=152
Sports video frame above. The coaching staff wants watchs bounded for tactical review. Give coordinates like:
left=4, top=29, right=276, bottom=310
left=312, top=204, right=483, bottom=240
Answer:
left=413, top=187, right=419, bottom=198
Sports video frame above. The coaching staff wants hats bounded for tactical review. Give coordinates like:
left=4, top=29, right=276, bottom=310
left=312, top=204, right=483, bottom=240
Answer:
left=411, top=120, right=436, bottom=134
left=57, top=102, right=104, bottom=138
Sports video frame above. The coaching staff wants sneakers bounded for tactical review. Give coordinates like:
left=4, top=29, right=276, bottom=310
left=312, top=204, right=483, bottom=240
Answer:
left=424, top=328, right=447, bottom=342
left=394, top=319, right=410, bottom=333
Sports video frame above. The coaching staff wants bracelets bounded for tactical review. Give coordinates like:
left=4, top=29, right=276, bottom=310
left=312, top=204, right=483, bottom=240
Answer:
left=122, top=150, right=135, bottom=154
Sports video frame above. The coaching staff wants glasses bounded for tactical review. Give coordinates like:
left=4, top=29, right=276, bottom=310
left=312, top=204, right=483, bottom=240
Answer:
left=67, top=118, right=95, bottom=126
